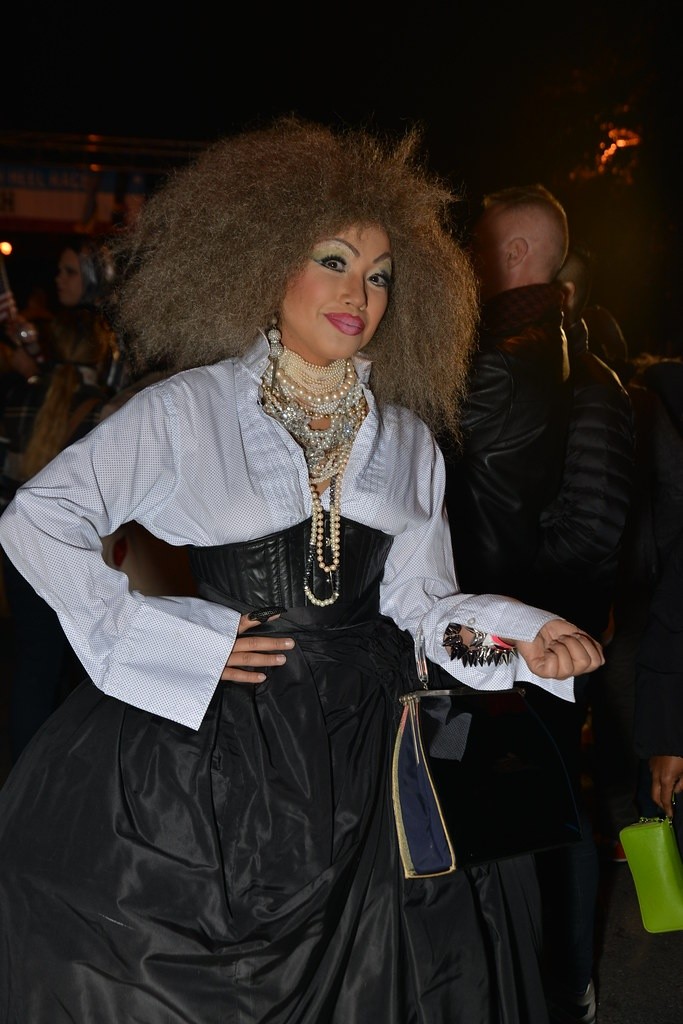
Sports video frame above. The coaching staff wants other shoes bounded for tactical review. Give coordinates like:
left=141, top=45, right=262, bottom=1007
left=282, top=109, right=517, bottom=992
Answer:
left=591, top=833, right=628, bottom=862
left=549, top=978, right=596, bottom=1024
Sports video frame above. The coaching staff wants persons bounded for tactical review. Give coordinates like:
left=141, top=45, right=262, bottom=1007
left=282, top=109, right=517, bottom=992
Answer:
left=1, top=124, right=607, bottom=1024
left=440, top=183, right=575, bottom=596
left=1, top=230, right=223, bottom=785
left=546, top=252, right=682, bottom=1022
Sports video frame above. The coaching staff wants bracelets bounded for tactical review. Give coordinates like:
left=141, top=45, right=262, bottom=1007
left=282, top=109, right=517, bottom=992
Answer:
left=442, top=622, right=519, bottom=668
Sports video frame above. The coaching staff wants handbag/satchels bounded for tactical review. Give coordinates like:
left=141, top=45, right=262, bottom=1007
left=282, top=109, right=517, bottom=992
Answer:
left=619, top=790, right=683, bottom=933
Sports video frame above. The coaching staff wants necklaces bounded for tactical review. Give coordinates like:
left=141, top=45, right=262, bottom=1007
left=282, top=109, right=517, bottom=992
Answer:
left=259, top=338, right=369, bottom=607
